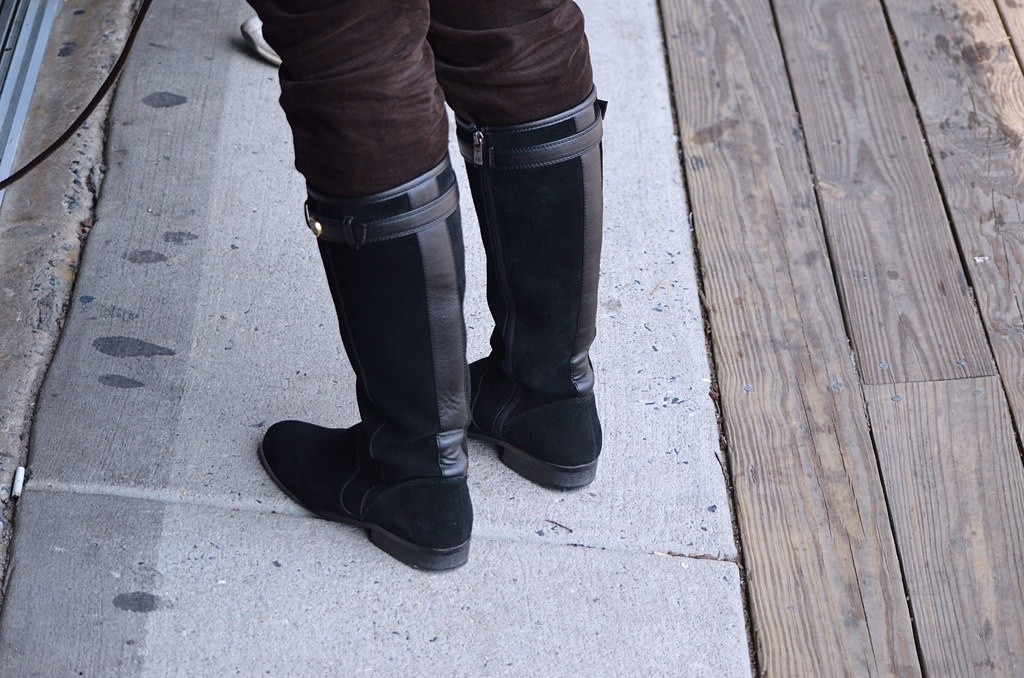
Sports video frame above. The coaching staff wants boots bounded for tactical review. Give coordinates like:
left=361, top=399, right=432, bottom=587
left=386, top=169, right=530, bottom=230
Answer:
left=257, top=155, right=474, bottom=571
left=456, top=81, right=608, bottom=488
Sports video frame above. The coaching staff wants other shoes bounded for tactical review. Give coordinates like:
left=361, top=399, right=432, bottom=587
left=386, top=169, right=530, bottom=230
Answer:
left=240, top=14, right=283, bottom=66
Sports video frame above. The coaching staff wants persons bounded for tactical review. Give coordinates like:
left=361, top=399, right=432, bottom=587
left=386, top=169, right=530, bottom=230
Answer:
left=241, top=1, right=608, bottom=571
